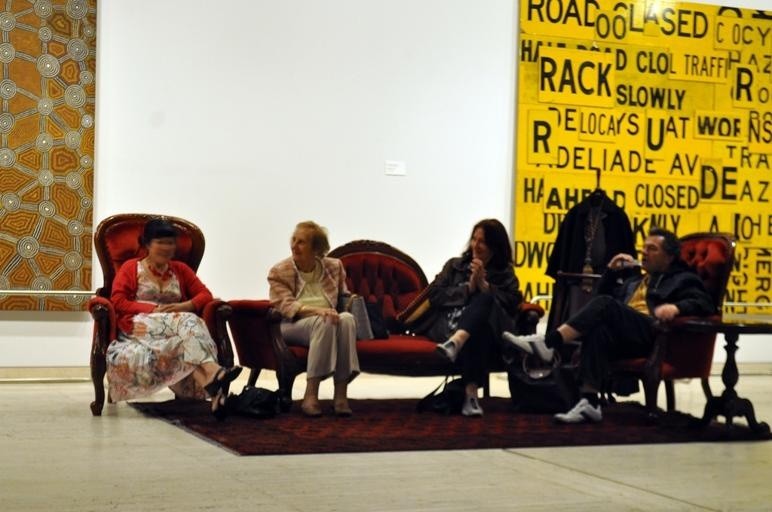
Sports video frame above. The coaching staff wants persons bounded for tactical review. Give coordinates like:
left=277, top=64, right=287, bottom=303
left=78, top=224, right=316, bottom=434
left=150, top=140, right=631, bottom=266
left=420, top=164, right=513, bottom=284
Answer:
left=502, top=228, right=716, bottom=424
left=268, top=221, right=360, bottom=416
left=106, top=220, right=242, bottom=422
left=428, top=219, right=523, bottom=418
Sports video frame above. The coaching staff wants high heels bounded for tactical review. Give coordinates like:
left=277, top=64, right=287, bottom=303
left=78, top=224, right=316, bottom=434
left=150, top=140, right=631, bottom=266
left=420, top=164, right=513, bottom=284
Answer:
left=204, top=366, right=243, bottom=422
left=334, top=399, right=353, bottom=415
left=302, top=400, right=323, bottom=415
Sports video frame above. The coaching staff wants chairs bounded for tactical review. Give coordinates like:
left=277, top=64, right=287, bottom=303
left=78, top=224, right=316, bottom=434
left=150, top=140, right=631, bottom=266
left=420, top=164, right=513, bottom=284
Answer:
left=87, top=212, right=235, bottom=417
left=596, top=231, right=736, bottom=417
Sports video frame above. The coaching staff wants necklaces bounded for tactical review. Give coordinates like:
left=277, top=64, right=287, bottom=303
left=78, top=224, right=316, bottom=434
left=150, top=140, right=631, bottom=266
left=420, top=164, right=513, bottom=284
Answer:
left=583, top=211, right=601, bottom=292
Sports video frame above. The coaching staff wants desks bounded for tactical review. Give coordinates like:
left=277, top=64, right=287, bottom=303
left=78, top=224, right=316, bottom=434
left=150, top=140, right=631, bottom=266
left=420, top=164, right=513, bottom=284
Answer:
left=674, top=315, right=772, bottom=435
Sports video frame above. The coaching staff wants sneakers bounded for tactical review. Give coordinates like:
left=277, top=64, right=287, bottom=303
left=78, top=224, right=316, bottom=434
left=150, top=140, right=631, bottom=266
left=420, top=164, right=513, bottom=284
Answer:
left=554, top=398, right=602, bottom=425
left=502, top=332, right=554, bottom=364
left=436, top=340, right=456, bottom=363
left=462, top=393, right=483, bottom=417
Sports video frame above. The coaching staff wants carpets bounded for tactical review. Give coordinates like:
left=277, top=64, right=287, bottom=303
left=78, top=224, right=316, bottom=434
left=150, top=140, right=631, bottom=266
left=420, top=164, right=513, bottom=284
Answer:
left=128, top=392, right=770, bottom=457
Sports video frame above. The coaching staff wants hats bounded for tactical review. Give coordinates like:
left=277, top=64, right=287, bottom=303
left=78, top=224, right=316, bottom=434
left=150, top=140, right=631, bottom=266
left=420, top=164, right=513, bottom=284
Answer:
left=143, top=220, right=179, bottom=242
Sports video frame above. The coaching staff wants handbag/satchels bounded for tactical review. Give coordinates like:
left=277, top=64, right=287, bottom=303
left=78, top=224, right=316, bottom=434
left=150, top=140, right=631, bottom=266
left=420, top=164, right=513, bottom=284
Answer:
left=340, top=294, right=374, bottom=339
left=236, top=386, right=283, bottom=419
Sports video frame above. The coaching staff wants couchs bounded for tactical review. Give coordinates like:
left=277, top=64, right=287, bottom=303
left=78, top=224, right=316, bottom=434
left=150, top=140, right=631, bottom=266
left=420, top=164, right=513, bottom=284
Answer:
left=228, top=237, right=545, bottom=413
